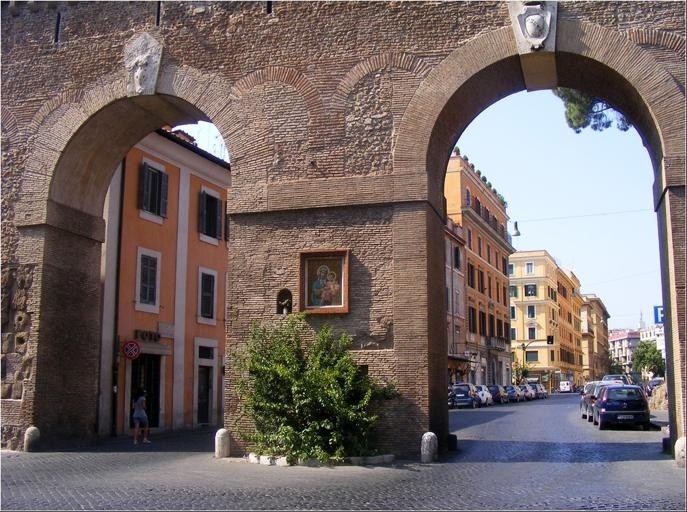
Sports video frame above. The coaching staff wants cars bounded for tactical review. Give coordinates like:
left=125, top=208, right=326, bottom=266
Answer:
left=646, top=376, right=664, bottom=397
left=576, top=385, right=584, bottom=393
left=581, top=373, right=650, bottom=431
left=445, top=382, right=548, bottom=409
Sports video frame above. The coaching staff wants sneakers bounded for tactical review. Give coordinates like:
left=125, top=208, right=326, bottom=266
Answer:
left=133, top=438, right=151, bottom=444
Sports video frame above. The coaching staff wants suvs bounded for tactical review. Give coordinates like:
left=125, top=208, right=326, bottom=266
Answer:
left=560, top=380, right=573, bottom=393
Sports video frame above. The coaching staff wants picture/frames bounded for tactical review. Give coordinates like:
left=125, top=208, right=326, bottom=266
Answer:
left=297, top=247, right=351, bottom=315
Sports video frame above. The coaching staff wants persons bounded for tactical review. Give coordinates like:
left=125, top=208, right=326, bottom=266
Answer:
left=131, top=386, right=152, bottom=446
left=310, top=265, right=340, bottom=306
